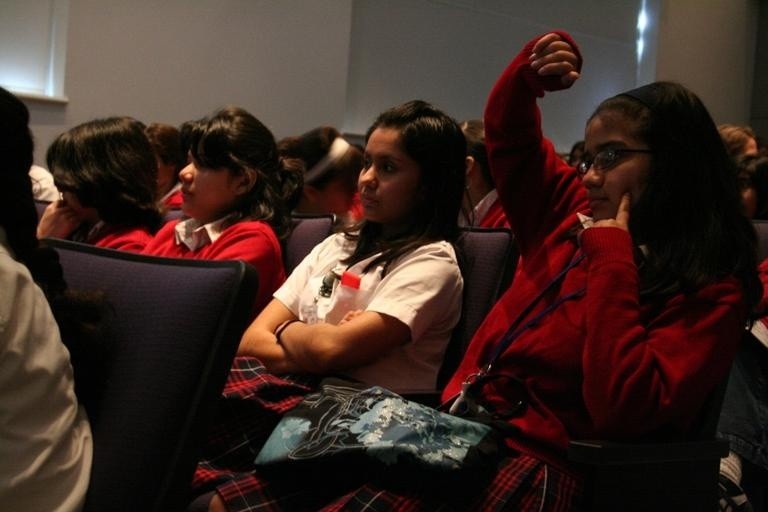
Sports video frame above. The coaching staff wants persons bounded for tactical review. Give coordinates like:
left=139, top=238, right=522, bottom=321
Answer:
left=189, top=28, right=760, bottom=511
left=1, top=88, right=512, bottom=509
left=718, top=124, right=768, bottom=221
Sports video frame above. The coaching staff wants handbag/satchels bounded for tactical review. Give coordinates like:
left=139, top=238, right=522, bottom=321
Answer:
left=254, top=378, right=504, bottom=495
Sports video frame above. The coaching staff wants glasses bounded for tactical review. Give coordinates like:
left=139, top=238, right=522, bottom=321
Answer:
left=577, top=148, right=651, bottom=176
